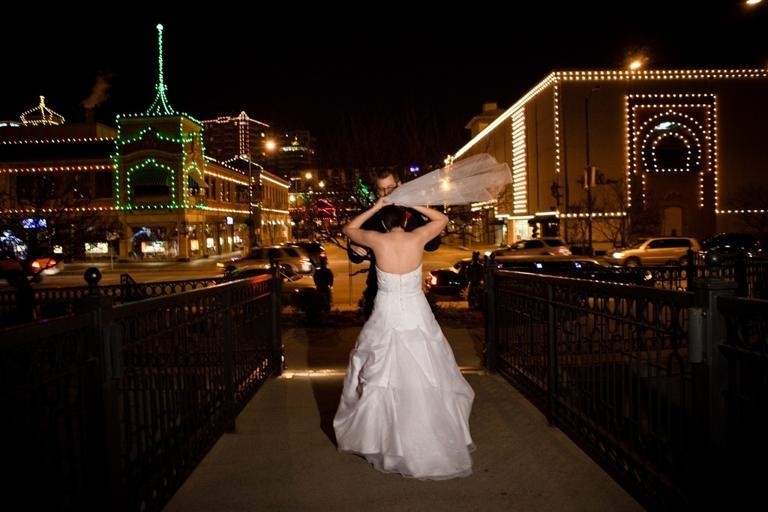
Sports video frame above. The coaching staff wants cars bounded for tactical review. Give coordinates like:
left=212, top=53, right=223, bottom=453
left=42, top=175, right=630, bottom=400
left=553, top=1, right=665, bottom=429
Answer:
left=0, top=236, right=60, bottom=286
left=491, top=238, right=571, bottom=255
left=211, top=241, right=327, bottom=296
left=424, top=255, right=653, bottom=305
left=620, top=233, right=760, bottom=267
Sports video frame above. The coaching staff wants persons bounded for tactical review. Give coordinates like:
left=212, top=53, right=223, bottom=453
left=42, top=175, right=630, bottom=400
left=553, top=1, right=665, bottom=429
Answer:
left=483, top=251, right=498, bottom=273
left=346, top=164, right=444, bottom=324
left=313, top=257, right=334, bottom=313
left=331, top=193, right=477, bottom=482
left=464, top=249, right=482, bottom=313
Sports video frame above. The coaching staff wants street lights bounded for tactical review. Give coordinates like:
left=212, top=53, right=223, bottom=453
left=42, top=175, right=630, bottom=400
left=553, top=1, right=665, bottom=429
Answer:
left=586, top=86, right=600, bottom=248
left=249, top=142, right=275, bottom=236
left=295, top=174, right=312, bottom=243
